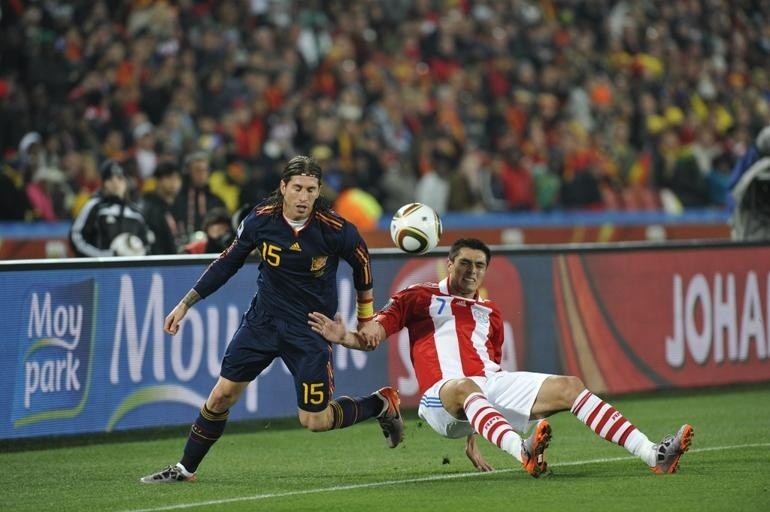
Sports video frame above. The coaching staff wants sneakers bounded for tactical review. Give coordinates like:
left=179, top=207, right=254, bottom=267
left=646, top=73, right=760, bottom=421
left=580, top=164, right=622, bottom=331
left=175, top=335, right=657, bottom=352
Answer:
left=519, top=418, right=552, bottom=479
left=139, top=464, right=198, bottom=483
left=371, top=385, right=404, bottom=449
left=649, top=422, right=694, bottom=476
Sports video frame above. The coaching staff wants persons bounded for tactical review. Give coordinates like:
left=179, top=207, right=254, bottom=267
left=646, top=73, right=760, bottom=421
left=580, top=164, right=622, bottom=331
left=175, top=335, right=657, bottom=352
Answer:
left=139, top=155, right=406, bottom=482
left=180, top=207, right=234, bottom=254
left=1, top=2, right=770, bottom=239
left=141, top=161, right=187, bottom=257
left=307, top=236, right=698, bottom=479
left=68, top=161, right=155, bottom=257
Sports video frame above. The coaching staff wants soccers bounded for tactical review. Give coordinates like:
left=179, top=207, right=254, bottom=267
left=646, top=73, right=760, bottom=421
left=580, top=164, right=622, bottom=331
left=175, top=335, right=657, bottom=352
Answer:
left=111, top=234, right=146, bottom=257
left=390, top=203, right=443, bottom=255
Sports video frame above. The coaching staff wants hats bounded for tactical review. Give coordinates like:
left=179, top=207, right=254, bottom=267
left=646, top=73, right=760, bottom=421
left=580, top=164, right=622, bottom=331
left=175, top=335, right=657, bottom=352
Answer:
left=100, top=159, right=130, bottom=183
